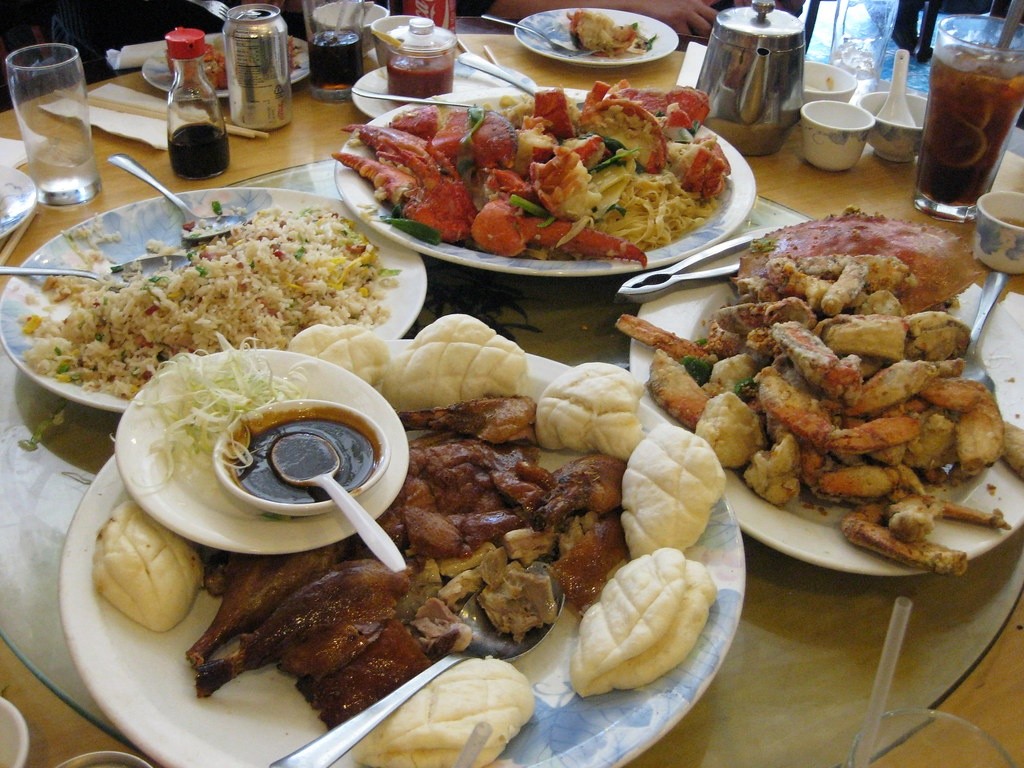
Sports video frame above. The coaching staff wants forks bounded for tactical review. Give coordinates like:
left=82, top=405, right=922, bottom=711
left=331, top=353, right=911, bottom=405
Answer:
left=481, top=14, right=599, bottom=57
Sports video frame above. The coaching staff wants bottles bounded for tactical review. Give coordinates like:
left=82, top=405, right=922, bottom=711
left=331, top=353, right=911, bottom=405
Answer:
left=164, top=26, right=230, bottom=181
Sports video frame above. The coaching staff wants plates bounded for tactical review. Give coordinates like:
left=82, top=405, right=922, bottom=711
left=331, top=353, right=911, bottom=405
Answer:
left=212, top=397, right=393, bottom=516
left=351, top=55, right=536, bottom=118
left=333, top=85, right=757, bottom=277
left=0, top=183, right=428, bottom=413
left=0, top=164, right=38, bottom=239
left=629, top=225, right=1024, bottom=577
left=57, top=336, right=748, bottom=768
left=0, top=697, right=30, bottom=768
left=141, top=32, right=310, bottom=98
left=113, top=347, right=411, bottom=556
left=514, top=7, right=679, bottom=68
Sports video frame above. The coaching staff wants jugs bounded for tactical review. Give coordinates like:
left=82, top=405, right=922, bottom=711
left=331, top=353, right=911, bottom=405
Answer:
left=693, top=1, right=806, bottom=157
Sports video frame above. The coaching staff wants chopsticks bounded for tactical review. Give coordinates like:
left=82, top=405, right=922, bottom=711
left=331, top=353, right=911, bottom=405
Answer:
left=53, top=87, right=269, bottom=140
left=188, top=0, right=230, bottom=22
left=0, top=202, right=37, bottom=269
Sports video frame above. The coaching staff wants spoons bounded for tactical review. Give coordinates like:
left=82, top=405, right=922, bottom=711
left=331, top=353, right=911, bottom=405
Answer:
left=267, top=563, right=566, bottom=768
left=0, top=254, right=192, bottom=293
left=271, top=431, right=406, bottom=573
left=108, top=153, right=246, bottom=241
left=876, top=48, right=916, bottom=127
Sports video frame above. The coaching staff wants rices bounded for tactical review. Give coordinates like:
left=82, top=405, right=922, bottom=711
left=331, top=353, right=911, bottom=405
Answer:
left=11, top=202, right=391, bottom=404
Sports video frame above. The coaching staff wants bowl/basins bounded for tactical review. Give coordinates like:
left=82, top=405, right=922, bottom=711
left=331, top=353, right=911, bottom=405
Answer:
left=385, top=16, right=458, bottom=108
left=370, top=15, right=422, bottom=66
left=312, top=0, right=390, bottom=58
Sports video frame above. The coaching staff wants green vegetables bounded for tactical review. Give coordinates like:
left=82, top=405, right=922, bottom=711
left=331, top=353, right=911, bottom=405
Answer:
left=380, top=106, right=699, bottom=243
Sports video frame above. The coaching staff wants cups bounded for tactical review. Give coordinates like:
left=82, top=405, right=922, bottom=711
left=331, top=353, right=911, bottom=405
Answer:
left=803, top=61, right=858, bottom=105
left=976, top=189, right=1024, bottom=276
left=856, top=91, right=929, bottom=163
left=800, top=99, right=876, bottom=172
left=6, top=42, right=103, bottom=209
left=302, top=0, right=365, bottom=104
left=847, top=707, right=1017, bottom=768
left=912, top=15, right=1024, bottom=224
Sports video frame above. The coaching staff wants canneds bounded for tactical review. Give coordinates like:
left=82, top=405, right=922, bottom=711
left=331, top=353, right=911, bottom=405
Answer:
left=221, top=4, right=291, bottom=132
left=403, top=0, right=457, bottom=34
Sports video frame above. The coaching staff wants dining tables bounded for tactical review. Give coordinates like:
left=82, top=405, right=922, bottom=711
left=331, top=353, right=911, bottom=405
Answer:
left=0, top=35, right=1024, bottom=768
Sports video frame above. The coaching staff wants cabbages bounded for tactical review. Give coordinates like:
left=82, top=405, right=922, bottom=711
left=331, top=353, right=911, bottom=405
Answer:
left=123, top=333, right=306, bottom=496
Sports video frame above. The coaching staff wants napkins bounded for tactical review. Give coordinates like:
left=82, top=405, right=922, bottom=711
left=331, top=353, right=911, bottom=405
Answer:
left=0, top=137, right=28, bottom=170
left=38, top=82, right=209, bottom=150
left=105, top=31, right=226, bottom=71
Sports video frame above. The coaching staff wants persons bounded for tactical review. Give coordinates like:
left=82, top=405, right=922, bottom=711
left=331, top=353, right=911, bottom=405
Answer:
left=0, top=0, right=307, bottom=114
left=486, top=0, right=718, bottom=42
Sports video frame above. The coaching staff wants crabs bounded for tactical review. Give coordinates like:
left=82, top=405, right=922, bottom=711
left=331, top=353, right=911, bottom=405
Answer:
left=616, top=209, right=1023, bottom=576
left=333, top=82, right=730, bottom=265
